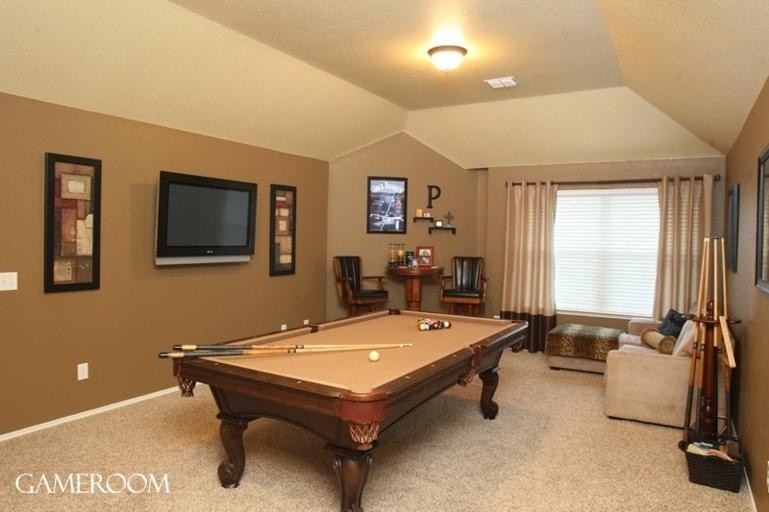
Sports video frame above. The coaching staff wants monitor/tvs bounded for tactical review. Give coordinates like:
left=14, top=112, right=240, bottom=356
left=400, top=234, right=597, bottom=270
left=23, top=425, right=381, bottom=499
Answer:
left=155, top=170, right=257, bottom=257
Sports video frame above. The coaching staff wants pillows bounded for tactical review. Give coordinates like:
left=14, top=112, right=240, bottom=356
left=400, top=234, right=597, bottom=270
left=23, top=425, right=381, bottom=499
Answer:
left=641, top=328, right=675, bottom=354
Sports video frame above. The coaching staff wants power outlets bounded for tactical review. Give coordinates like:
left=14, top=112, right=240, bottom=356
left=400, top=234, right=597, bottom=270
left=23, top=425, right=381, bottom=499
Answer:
left=0, top=272, right=18, bottom=291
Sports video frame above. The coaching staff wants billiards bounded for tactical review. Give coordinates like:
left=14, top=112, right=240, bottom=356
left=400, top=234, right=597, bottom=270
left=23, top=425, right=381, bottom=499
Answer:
left=418, top=317, right=451, bottom=330
left=369, top=351, right=380, bottom=361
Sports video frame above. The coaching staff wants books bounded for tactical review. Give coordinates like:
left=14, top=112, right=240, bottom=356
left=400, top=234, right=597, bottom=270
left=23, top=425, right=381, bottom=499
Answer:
left=680, top=425, right=736, bottom=465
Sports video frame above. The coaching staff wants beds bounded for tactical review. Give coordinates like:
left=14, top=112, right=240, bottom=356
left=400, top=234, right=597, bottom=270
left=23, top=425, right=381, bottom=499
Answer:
left=545, top=317, right=696, bottom=374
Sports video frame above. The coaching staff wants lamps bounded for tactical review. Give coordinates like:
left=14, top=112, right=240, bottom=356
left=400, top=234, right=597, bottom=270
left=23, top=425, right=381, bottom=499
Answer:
left=427, top=45, right=468, bottom=75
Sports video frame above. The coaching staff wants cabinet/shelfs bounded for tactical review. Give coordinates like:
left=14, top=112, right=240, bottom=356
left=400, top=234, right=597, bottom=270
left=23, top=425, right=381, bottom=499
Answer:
left=412, top=216, right=456, bottom=234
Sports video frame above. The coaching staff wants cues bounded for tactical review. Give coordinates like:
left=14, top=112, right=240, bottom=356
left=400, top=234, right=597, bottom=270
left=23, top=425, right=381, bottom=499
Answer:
left=159, top=344, right=414, bottom=359
left=682, top=237, right=735, bottom=443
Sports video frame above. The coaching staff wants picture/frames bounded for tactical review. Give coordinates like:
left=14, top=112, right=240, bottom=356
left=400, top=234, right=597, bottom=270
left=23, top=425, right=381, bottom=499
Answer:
left=45, top=152, right=102, bottom=293
left=367, top=175, right=407, bottom=234
left=727, top=183, right=739, bottom=273
left=270, top=184, right=296, bottom=276
left=416, top=246, right=434, bottom=266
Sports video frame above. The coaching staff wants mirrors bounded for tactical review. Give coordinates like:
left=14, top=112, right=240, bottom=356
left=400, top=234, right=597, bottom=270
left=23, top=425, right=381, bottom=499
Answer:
left=755, top=145, right=769, bottom=293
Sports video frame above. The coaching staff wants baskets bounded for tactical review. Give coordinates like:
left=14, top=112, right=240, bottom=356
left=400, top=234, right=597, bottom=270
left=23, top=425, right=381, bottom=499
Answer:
left=685, top=435, right=744, bottom=493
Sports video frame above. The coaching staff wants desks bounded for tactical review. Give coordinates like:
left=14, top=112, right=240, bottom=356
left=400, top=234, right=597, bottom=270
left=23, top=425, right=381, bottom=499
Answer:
left=173, top=308, right=529, bottom=512
left=386, top=265, right=443, bottom=312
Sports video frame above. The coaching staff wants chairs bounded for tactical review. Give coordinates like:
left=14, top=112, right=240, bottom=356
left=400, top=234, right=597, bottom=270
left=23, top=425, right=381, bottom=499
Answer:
left=333, top=256, right=387, bottom=317
left=605, top=322, right=726, bottom=433
left=440, top=255, right=488, bottom=316
